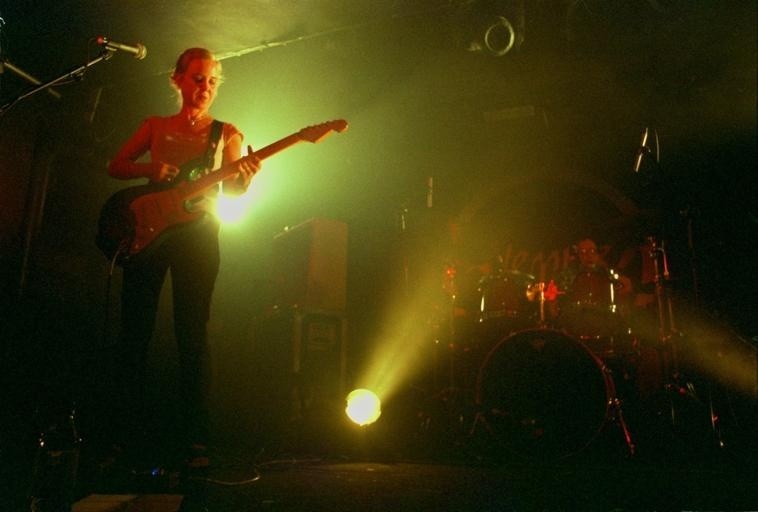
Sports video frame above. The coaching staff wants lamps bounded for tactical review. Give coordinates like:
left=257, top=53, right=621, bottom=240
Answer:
left=467, top=12, right=515, bottom=57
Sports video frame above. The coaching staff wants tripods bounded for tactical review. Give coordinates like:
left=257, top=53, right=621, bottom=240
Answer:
left=597, top=358, right=636, bottom=456
left=639, top=239, right=724, bottom=447
left=415, top=281, right=481, bottom=430
left=593, top=273, right=654, bottom=411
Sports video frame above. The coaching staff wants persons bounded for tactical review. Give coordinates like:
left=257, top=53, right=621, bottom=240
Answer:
left=526, top=237, right=633, bottom=306
left=97, top=48, right=260, bottom=469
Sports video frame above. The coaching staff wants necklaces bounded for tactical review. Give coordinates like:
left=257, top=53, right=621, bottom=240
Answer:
left=189, top=114, right=209, bottom=126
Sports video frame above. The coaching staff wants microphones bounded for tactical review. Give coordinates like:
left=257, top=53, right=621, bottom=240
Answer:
left=88, top=84, right=104, bottom=120
left=96, top=32, right=149, bottom=63
left=632, top=119, right=660, bottom=178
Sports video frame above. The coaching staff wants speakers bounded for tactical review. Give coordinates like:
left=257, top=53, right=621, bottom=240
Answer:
left=264, top=217, right=350, bottom=304
left=256, top=303, right=349, bottom=458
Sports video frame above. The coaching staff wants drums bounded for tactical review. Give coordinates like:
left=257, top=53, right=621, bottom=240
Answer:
left=474, top=270, right=537, bottom=325
left=479, top=331, right=614, bottom=464
left=552, top=271, right=622, bottom=337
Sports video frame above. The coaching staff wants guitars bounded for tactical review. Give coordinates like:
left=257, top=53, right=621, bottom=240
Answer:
left=96, top=119, right=347, bottom=265
